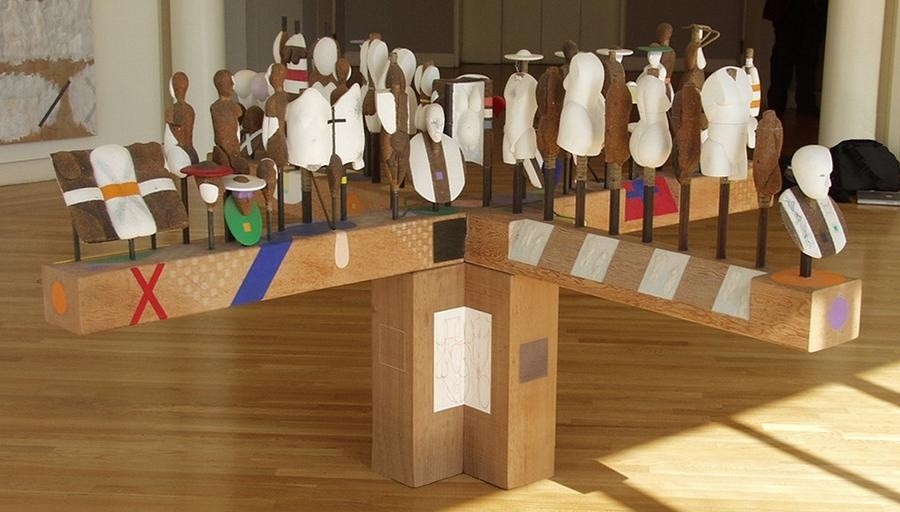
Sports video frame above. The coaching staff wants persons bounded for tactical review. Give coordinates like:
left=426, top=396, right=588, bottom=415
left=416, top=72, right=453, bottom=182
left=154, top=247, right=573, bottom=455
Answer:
left=759, top=1, right=830, bottom=123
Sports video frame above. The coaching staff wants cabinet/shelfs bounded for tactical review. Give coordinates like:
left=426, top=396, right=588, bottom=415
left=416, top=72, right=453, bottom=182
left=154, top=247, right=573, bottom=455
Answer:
left=459, top=0, right=626, bottom=66
